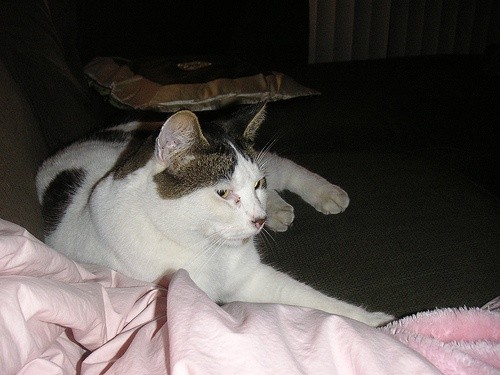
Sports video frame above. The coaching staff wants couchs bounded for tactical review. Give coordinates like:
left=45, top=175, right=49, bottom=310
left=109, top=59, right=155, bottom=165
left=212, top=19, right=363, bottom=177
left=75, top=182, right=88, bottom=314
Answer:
left=0, top=0, right=500, bottom=318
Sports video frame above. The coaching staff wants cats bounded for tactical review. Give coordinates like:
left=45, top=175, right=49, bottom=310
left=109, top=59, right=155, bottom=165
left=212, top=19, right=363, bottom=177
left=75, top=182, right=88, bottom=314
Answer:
left=34, top=108, right=399, bottom=328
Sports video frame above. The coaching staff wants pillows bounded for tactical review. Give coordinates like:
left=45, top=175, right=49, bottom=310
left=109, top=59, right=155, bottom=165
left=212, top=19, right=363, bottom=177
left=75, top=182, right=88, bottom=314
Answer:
left=84, top=53, right=323, bottom=115
